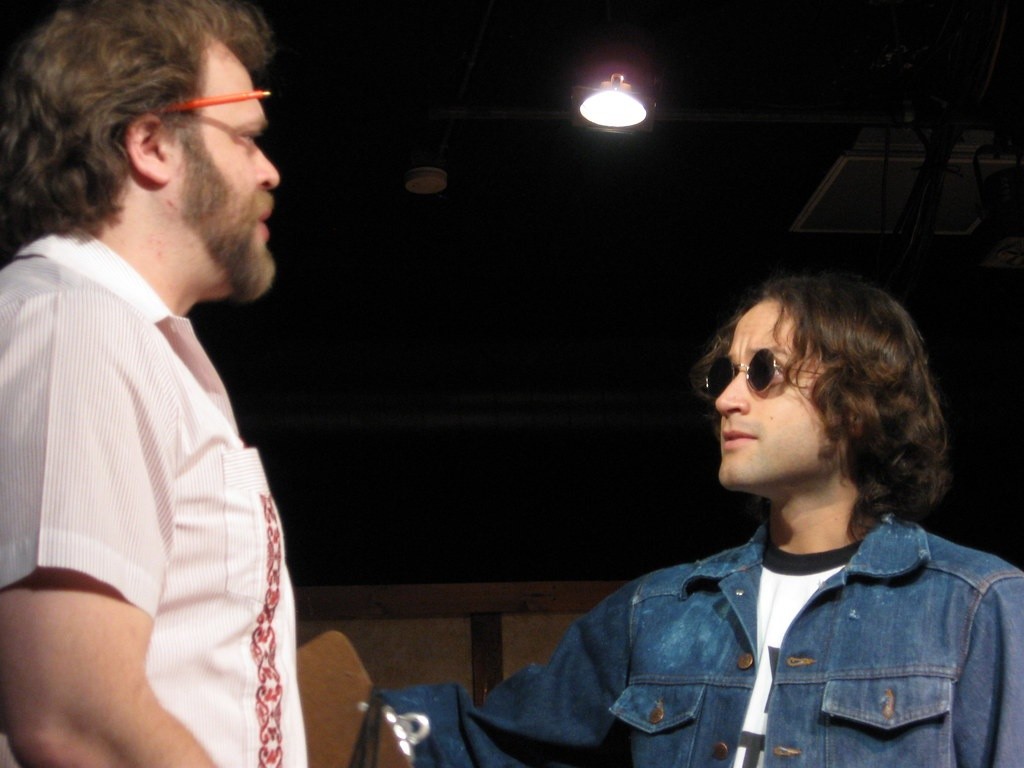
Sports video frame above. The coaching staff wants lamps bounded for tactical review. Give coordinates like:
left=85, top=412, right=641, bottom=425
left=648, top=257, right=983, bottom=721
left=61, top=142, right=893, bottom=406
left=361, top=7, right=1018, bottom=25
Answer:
left=569, top=73, right=656, bottom=135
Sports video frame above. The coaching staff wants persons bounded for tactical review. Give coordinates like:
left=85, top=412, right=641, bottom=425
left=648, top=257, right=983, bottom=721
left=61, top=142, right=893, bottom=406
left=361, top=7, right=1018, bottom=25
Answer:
left=369, top=278, right=1024, bottom=768
left=0, top=0, right=309, bottom=768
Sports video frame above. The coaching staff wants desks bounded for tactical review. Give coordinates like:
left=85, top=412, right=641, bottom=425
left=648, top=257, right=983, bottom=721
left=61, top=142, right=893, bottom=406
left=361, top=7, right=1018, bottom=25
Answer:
left=296, top=578, right=629, bottom=702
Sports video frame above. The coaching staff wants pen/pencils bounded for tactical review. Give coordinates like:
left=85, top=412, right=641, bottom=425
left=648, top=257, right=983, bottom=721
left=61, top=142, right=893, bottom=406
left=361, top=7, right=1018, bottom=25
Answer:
left=149, top=90, right=271, bottom=112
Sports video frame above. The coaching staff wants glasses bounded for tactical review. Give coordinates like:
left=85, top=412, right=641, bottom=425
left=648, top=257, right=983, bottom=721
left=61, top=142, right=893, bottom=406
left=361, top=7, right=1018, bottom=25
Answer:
left=706, top=347, right=824, bottom=398
